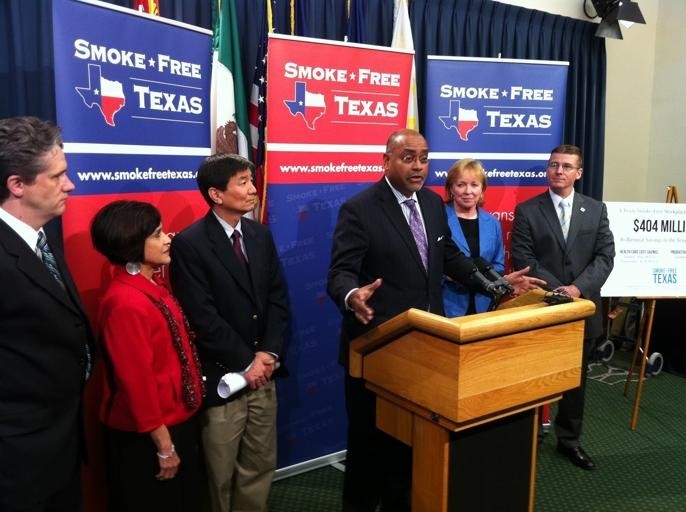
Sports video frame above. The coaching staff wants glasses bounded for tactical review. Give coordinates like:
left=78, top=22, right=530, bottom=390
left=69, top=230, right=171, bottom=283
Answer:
left=547, top=162, right=580, bottom=171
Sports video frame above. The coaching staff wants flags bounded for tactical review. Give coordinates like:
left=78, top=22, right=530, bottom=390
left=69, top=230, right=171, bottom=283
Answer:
left=340, top=0, right=369, bottom=45
left=204, top=0, right=259, bottom=216
left=285, top=0, right=309, bottom=38
left=390, top=0, right=421, bottom=132
left=134, top=0, right=162, bottom=17
left=246, top=0, right=282, bottom=223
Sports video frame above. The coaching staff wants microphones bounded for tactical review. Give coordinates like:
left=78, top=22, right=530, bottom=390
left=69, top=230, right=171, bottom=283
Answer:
left=473, top=256, right=515, bottom=295
left=459, top=257, right=506, bottom=298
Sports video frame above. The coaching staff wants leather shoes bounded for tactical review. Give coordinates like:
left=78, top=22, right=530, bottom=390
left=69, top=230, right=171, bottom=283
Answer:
left=556, top=440, right=596, bottom=470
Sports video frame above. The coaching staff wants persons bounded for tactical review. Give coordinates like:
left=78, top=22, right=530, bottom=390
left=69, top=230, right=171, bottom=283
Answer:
left=0, top=114, right=102, bottom=512
left=444, top=158, right=506, bottom=321
left=89, top=199, right=212, bottom=510
left=513, top=142, right=614, bottom=470
left=169, top=153, right=293, bottom=512
left=327, top=127, right=548, bottom=512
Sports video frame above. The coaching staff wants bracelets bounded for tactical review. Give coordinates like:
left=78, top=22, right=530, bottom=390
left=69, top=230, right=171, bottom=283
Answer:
left=156, top=443, right=177, bottom=460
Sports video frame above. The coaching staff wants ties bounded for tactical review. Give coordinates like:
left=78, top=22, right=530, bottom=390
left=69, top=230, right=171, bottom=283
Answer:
left=405, top=199, right=430, bottom=312
left=560, top=200, right=572, bottom=242
left=232, top=230, right=246, bottom=267
left=36, top=230, right=68, bottom=294
